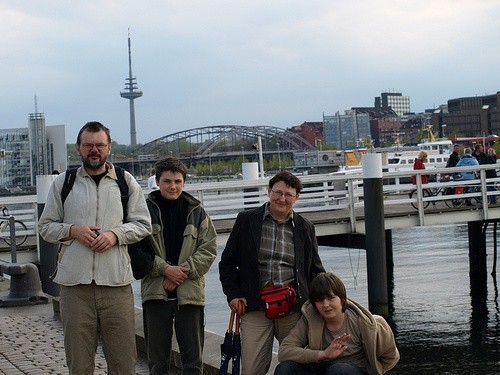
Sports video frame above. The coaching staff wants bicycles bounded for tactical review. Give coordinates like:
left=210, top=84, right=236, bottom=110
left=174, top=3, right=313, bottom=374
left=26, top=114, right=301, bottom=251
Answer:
left=0, top=204, right=28, bottom=247
left=408, top=170, right=500, bottom=210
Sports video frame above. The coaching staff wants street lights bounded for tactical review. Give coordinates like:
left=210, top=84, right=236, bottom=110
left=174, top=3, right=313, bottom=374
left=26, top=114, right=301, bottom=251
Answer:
left=241, top=145, right=244, bottom=162
left=130, top=152, right=134, bottom=174
left=276, top=142, right=282, bottom=170
left=441, top=124, right=446, bottom=138
left=207, top=149, right=212, bottom=176
left=169, top=150, right=172, bottom=156
left=111, top=153, right=115, bottom=163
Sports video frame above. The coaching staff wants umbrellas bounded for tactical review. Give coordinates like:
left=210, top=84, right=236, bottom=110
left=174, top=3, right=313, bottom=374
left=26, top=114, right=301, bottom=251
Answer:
left=217, top=300, right=242, bottom=375
left=231, top=299, right=247, bottom=375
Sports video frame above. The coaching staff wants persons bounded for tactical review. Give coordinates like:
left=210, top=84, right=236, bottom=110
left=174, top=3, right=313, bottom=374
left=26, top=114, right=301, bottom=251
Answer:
left=39, top=121, right=153, bottom=375
left=273, top=273, right=400, bottom=375
left=127, top=156, right=218, bottom=375
left=218, top=171, right=327, bottom=375
left=412, top=143, right=499, bottom=206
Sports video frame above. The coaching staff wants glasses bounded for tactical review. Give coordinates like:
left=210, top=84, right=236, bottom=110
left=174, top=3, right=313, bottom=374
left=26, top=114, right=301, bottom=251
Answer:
left=271, top=190, right=295, bottom=198
left=80, top=143, right=109, bottom=149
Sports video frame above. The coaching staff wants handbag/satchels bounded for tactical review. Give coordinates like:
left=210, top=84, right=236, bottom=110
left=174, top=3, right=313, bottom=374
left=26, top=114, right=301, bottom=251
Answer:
left=258, top=284, right=298, bottom=320
